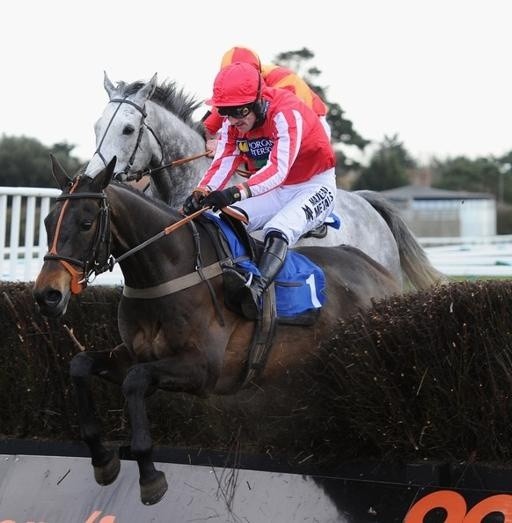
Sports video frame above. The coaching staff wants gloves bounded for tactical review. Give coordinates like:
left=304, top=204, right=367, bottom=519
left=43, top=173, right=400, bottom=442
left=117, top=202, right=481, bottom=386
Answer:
left=200, top=186, right=241, bottom=212
left=206, top=139, right=221, bottom=157
left=182, top=190, right=204, bottom=217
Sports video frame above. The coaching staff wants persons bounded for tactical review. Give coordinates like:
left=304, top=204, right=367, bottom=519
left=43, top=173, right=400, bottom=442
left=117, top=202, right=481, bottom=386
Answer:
left=182, top=60, right=340, bottom=322
left=197, top=46, right=332, bottom=159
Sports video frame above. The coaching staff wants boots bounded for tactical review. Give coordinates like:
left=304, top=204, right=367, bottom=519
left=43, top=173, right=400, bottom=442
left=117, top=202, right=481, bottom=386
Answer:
left=227, top=231, right=289, bottom=320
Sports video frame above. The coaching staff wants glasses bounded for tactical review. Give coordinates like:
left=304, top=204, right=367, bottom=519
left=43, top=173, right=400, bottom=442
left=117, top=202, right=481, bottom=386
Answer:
left=218, top=105, right=252, bottom=118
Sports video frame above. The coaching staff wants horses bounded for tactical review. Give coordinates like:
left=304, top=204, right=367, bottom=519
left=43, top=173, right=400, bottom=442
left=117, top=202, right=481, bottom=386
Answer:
left=32, top=154, right=403, bottom=506
left=84, top=70, right=450, bottom=294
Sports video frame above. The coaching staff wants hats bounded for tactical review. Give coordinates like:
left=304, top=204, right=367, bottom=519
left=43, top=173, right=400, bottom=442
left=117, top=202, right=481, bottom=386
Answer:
left=220, top=48, right=262, bottom=74
left=205, top=61, right=266, bottom=107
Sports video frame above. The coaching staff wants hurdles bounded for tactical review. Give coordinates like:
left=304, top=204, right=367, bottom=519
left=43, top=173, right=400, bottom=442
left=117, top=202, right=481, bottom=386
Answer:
left=1, top=280, right=512, bottom=522
